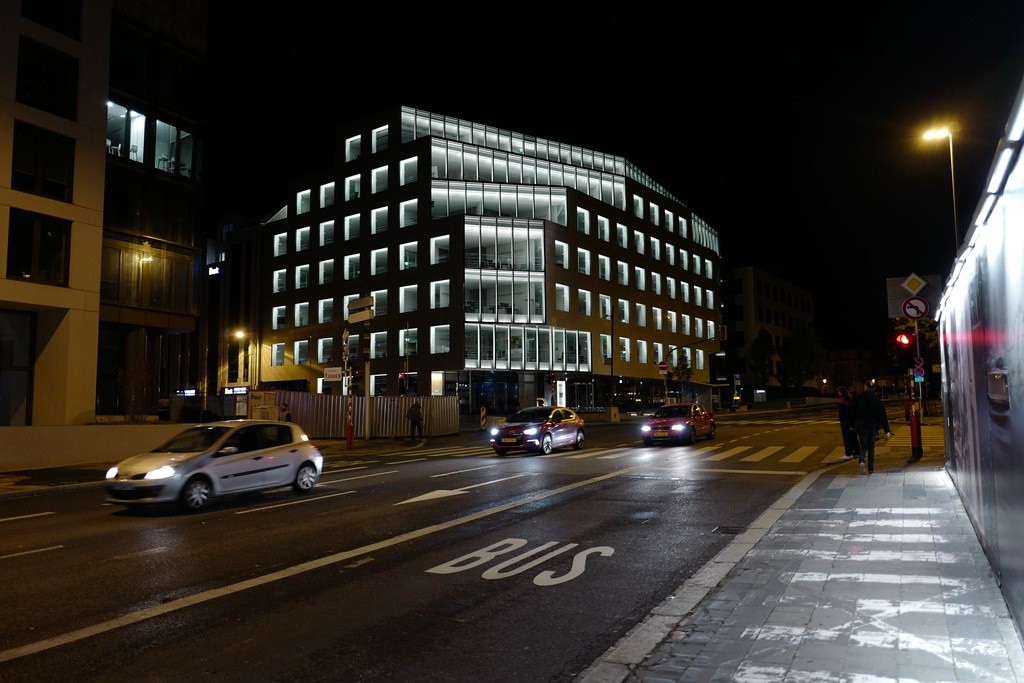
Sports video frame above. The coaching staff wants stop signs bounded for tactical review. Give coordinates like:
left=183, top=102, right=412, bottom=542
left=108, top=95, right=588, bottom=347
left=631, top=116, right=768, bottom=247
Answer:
left=659, top=361, right=668, bottom=370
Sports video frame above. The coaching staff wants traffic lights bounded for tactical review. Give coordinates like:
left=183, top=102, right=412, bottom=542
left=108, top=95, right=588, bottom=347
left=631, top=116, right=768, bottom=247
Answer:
left=550, top=372, right=556, bottom=384
left=897, top=331, right=913, bottom=347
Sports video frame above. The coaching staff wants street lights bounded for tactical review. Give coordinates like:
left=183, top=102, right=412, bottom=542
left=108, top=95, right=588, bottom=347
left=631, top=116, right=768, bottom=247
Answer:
left=611, top=304, right=614, bottom=407
left=664, top=337, right=718, bottom=404
left=923, top=128, right=958, bottom=252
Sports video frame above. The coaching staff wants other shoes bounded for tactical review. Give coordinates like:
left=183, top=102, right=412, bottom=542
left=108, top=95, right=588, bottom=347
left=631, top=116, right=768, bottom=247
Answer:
left=869, top=471, right=875, bottom=475
left=837, top=456, right=851, bottom=461
left=860, top=462, right=866, bottom=474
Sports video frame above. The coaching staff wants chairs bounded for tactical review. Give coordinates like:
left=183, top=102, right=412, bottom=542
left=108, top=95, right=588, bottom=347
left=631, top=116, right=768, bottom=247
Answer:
left=106, top=139, right=173, bottom=173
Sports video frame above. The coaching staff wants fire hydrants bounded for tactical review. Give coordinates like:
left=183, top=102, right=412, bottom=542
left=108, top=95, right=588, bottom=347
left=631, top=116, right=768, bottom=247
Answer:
left=345, top=422, right=354, bottom=449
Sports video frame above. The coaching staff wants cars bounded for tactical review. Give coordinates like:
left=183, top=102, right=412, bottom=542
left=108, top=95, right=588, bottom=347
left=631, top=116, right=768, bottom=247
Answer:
left=104, top=419, right=324, bottom=511
left=490, top=406, right=585, bottom=455
left=642, top=403, right=715, bottom=446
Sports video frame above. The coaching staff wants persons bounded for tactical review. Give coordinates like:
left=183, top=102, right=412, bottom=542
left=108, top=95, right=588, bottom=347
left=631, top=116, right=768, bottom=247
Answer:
left=837, top=387, right=860, bottom=459
left=409, top=401, right=424, bottom=440
left=278, top=403, right=291, bottom=422
left=487, top=348, right=492, bottom=360
left=847, top=382, right=891, bottom=476
left=712, top=399, right=719, bottom=413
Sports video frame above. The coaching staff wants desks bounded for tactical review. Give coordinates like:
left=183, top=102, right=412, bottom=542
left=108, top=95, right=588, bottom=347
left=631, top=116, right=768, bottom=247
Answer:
left=716, top=408, right=723, bottom=414
